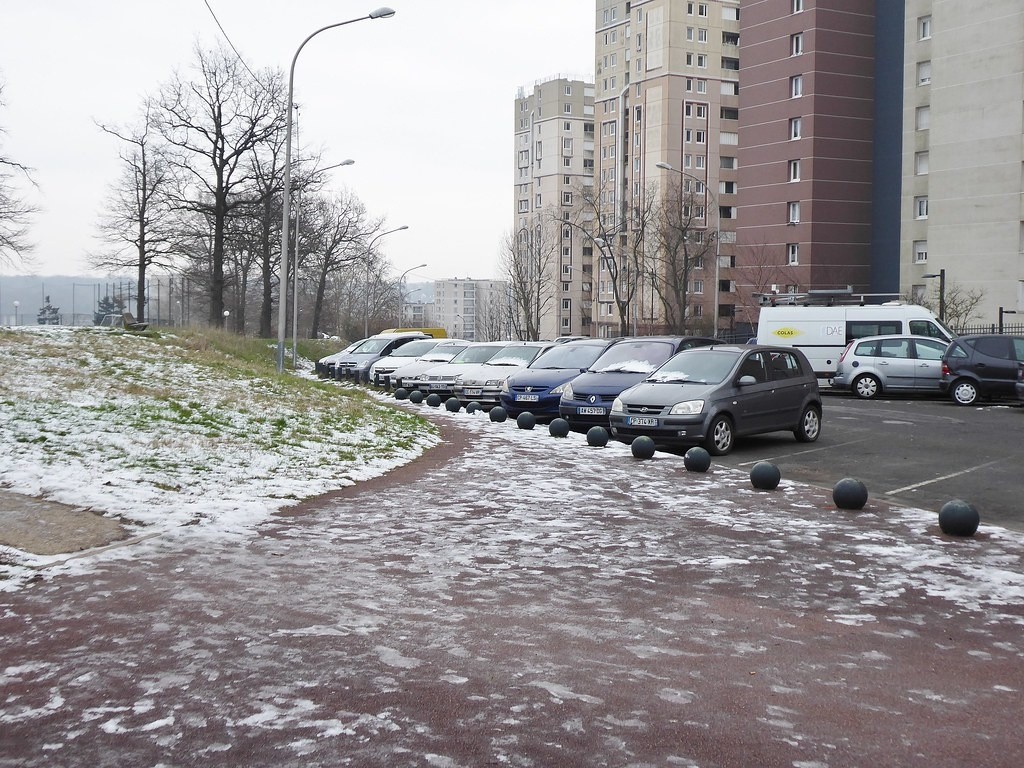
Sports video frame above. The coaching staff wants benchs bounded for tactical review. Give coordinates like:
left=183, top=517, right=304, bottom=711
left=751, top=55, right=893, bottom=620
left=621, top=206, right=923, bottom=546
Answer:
left=122, top=313, right=150, bottom=331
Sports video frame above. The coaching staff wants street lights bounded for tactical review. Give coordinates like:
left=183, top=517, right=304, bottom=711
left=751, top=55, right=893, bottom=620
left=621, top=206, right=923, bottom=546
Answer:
left=540, top=277, right=563, bottom=336
left=277, top=6, right=395, bottom=373
left=456, top=314, right=466, bottom=338
left=655, top=161, right=720, bottom=341
left=292, top=158, right=355, bottom=365
left=595, top=237, right=637, bottom=336
left=223, top=310, right=229, bottom=332
left=364, top=226, right=427, bottom=339
left=921, top=274, right=1017, bottom=335
left=567, top=265, right=598, bottom=336
left=13, top=301, right=20, bottom=326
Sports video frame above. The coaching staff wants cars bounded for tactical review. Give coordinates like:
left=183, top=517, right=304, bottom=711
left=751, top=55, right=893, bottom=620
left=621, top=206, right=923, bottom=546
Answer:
left=452, top=341, right=563, bottom=411
left=609, top=344, right=823, bottom=456
left=325, top=335, right=375, bottom=368
left=390, top=342, right=479, bottom=391
left=559, top=335, right=727, bottom=430
left=319, top=338, right=365, bottom=365
left=939, top=334, right=1024, bottom=407
left=370, top=339, right=470, bottom=381
left=335, top=331, right=432, bottom=379
left=499, top=336, right=635, bottom=419
left=828, top=334, right=967, bottom=400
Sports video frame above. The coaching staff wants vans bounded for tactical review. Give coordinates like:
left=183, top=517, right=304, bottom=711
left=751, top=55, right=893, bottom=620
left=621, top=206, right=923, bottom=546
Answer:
left=379, top=328, right=447, bottom=339
left=751, top=284, right=966, bottom=392
